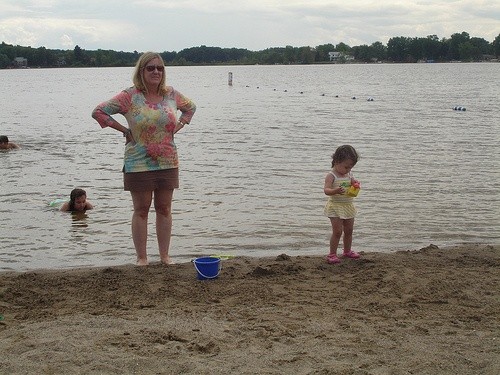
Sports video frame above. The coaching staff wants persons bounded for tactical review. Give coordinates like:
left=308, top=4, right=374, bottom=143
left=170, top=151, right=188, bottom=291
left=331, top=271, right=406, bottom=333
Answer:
left=0, top=135, right=19, bottom=148
left=59, top=189, right=93, bottom=210
left=91, top=52, right=197, bottom=265
left=322, top=145, right=361, bottom=263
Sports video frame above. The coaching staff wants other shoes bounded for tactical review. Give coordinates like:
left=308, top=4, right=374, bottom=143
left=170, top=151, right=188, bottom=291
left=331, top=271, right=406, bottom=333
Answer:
left=342, top=250, right=360, bottom=259
left=328, top=253, right=341, bottom=265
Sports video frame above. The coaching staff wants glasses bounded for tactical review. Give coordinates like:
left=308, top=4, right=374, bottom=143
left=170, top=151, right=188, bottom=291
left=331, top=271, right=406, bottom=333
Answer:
left=143, top=65, right=164, bottom=72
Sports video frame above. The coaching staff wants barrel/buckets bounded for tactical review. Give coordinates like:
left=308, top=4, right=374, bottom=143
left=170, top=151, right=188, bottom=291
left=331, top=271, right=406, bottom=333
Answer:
left=193, top=257, right=222, bottom=280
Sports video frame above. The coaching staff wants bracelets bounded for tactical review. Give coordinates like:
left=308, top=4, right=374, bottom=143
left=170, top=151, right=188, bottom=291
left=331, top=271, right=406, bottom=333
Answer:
left=178, top=120, right=183, bottom=125
left=123, top=130, right=130, bottom=137
